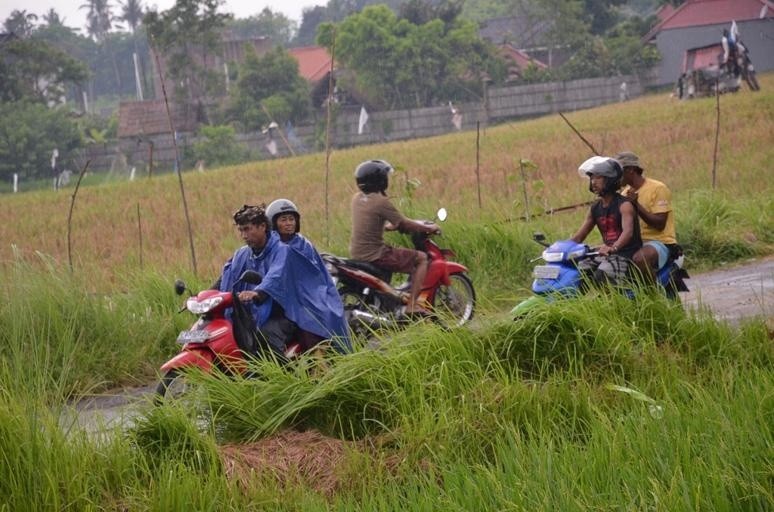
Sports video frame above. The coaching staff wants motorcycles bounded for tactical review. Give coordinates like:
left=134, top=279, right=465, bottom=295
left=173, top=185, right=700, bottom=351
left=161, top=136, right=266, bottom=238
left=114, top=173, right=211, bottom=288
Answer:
left=511, top=232, right=690, bottom=322
left=152, top=270, right=311, bottom=412
left=319, top=207, right=477, bottom=348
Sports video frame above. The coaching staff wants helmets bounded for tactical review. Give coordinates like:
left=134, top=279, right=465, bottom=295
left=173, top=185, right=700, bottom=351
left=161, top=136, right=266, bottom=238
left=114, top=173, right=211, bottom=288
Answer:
left=578, top=151, right=644, bottom=193
left=265, top=199, right=300, bottom=232
left=354, top=160, right=394, bottom=194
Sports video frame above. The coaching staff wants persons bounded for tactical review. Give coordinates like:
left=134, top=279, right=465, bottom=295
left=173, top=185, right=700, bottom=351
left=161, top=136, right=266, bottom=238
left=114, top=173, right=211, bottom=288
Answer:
left=350, top=159, right=442, bottom=315
left=210, top=198, right=350, bottom=369
left=568, top=152, right=678, bottom=284
